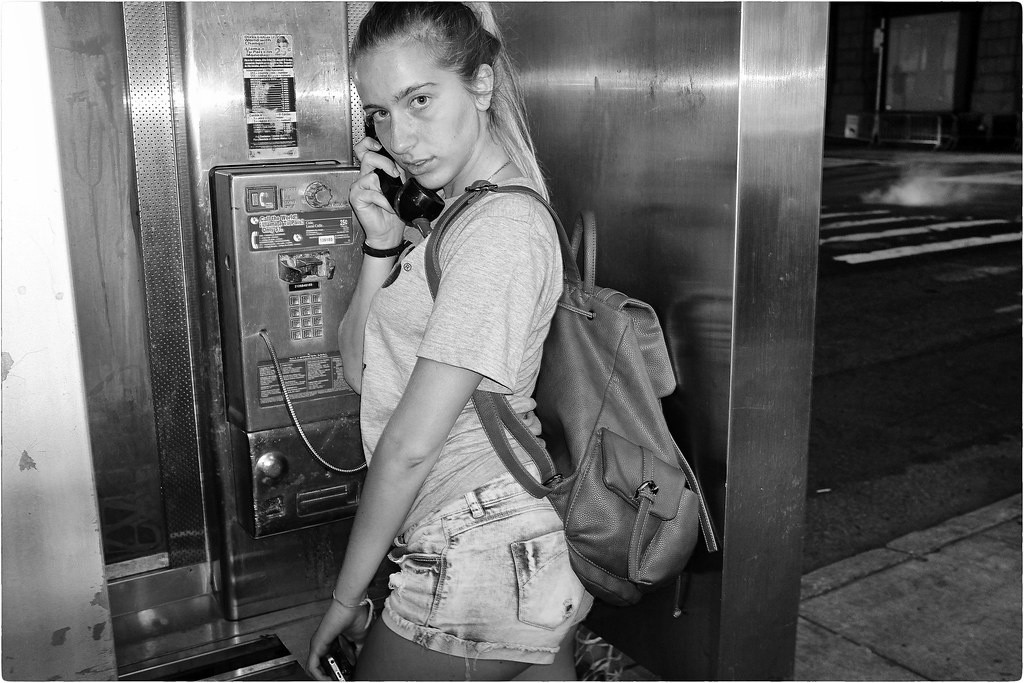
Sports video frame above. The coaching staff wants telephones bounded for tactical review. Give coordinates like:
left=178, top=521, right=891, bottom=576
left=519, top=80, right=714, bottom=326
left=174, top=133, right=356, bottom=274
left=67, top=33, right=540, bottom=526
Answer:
left=358, top=111, right=446, bottom=228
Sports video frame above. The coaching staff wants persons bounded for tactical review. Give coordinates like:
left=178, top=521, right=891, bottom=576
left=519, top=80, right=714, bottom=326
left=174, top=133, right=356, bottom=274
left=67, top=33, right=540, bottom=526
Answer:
left=306, top=2, right=593, bottom=683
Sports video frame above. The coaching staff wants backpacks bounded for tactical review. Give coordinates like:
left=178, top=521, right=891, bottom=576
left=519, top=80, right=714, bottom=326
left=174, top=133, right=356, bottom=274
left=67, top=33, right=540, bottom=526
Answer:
left=426, top=179, right=718, bottom=625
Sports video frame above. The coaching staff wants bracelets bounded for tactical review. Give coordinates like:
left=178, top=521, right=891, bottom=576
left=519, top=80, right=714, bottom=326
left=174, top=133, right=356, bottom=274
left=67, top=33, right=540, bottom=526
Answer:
left=333, top=588, right=374, bottom=630
